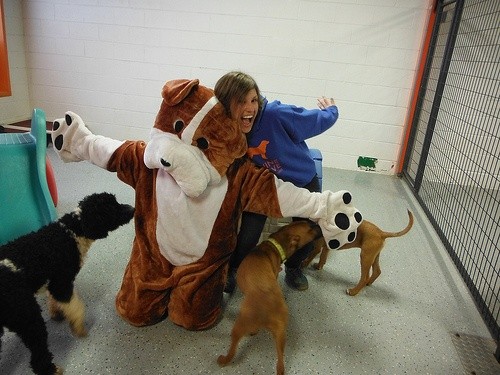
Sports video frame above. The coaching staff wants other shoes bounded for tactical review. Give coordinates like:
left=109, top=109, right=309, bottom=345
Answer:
left=285, top=266, right=308, bottom=291
left=223, top=271, right=237, bottom=293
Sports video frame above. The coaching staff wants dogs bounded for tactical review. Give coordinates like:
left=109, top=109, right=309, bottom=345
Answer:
left=216, top=208, right=415, bottom=375
left=0, top=190, right=134, bottom=375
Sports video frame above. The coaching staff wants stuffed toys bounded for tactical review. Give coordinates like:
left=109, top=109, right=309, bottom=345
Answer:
left=51, top=77, right=363, bottom=330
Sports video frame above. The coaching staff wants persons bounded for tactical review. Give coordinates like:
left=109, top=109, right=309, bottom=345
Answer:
left=214, top=71, right=339, bottom=294
